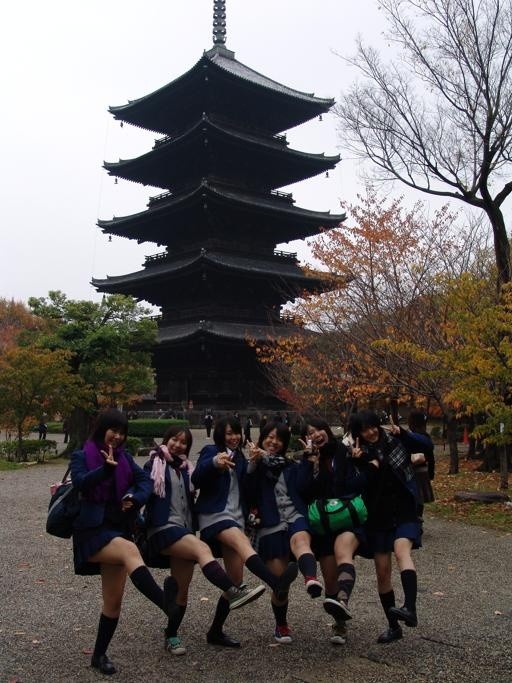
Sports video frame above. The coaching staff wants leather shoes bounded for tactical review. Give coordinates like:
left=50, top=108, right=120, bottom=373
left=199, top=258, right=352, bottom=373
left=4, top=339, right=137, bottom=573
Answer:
left=206, top=631, right=240, bottom=648
left=164, top=577, right=177, bottom=616
left=275, top=562, right=298, bottom=602
left=91, top=653, right=116, bottom=675
left=378, top=628, right=402, bottom=643
left=390, top=607, right=417, bottom=627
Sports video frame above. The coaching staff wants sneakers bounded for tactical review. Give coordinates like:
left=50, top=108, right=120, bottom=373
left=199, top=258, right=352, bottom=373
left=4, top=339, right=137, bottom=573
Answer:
left=274, top=624, right=292, bottom=643
left=324, top=599, right=352, bottom=621
left=330, top=623, right=346, bottom=644
left=305, top=575, right=322, bottom=598
left=224, top=585, right=265, bottom=610
left=164, top=636, right=186, bottom=655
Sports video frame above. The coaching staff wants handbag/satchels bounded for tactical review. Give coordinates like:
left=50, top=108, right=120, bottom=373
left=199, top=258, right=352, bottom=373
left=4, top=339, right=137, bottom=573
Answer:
left=46, top=484, right=81, bottom=539
left=308, top=496, right=369, bottom=534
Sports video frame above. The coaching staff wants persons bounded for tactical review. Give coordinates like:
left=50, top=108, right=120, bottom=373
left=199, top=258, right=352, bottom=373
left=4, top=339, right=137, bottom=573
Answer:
left=126, top=408, right=138, bottom=420
left=206, top=412, right=291, bottom=444
left=62, top=416, right=69, bottom=442
left=141, top=425, right=266, bottom=655
left=295, top=416, right=369, bottom=645
left=407, top=410, right=435, bottom=535
left=248, top=422, right=325, bottom=644
left=348, top=408, right=434, bottom=644
left=191, top=416, right=299, bottom=647
left=46, top=410, right=180, bottom=676
left=39, top=418, right=48, bottom=441
